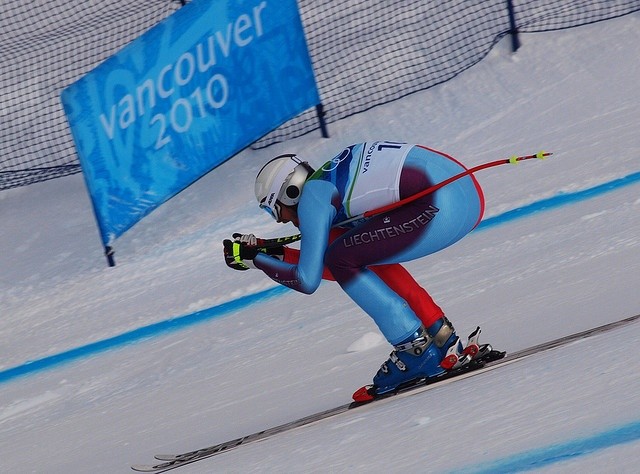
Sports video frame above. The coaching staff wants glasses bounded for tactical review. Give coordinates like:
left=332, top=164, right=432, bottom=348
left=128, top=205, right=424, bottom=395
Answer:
left=260, top=156, right=305, bottom=223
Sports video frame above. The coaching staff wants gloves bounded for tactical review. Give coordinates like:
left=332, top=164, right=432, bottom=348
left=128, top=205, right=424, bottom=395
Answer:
left=224, top=240, right=260, bottom=271
left=234, top=233, right=258, bottom=248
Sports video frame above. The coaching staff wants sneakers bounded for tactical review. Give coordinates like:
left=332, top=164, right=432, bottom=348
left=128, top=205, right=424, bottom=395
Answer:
left=428, top=316, right=464, bottom=358
left=374, top=324, right=444, bottom=387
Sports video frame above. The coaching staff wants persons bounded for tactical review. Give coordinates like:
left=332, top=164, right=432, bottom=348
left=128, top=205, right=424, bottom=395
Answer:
left=223, top=140, right=485, bottom=390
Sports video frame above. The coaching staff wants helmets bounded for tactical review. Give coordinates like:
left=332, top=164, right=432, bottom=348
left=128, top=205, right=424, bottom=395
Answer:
left=255, top=154, right=316, bottom=207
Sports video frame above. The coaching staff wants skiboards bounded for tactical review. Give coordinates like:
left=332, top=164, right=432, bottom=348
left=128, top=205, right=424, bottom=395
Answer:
left=131, top=315, right=640, bottom=473
